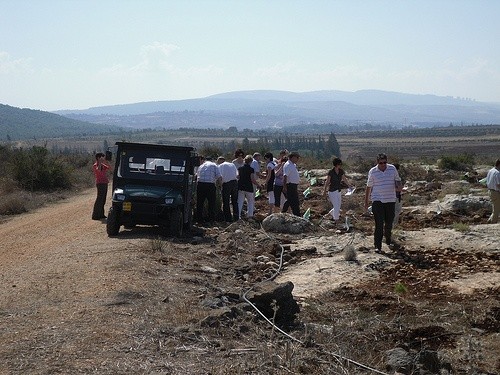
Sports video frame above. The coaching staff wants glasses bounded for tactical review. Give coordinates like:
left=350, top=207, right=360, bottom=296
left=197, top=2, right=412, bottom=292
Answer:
left=378, top=161, right=387, bottom=165
left=337, top=164, right=342, bottom=165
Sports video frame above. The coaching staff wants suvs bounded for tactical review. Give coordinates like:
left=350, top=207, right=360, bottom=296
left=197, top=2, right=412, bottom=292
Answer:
left=104, top=141, right=197, bottom=238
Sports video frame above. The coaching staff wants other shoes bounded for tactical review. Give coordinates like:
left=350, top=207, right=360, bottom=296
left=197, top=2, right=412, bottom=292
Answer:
left=323, top=215, right=332, bottom=219
left=101, top=215, right=107, bottom=218
left=386, top=238, right=392, bottom=245
left=375, top=247, right=381, bottom=253
left=92, top=216, right=102, bottom=220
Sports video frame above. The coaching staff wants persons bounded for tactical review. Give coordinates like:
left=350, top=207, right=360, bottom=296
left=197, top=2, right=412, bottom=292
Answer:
left=196, top=156, right=220, bottom=225
left=274, top=156, right=290, bottom=213
left=322, top=158, right=355, bottom=223
left=283, top=151, right=301, bottom=217
left=92, top=152, right=112, bottom=220
left=232, top=148, right=264, bottom=213
left=392, top=166, right=406, bottom=228
left=486, top=158, right=500, bottom=224
left=237, top=154, right=258, bottom=222
left=364, top=153, right=410, bottom=253
left=263, top=152, right=276, bottom=216
left=217, top=157, right=239, bottom=223
